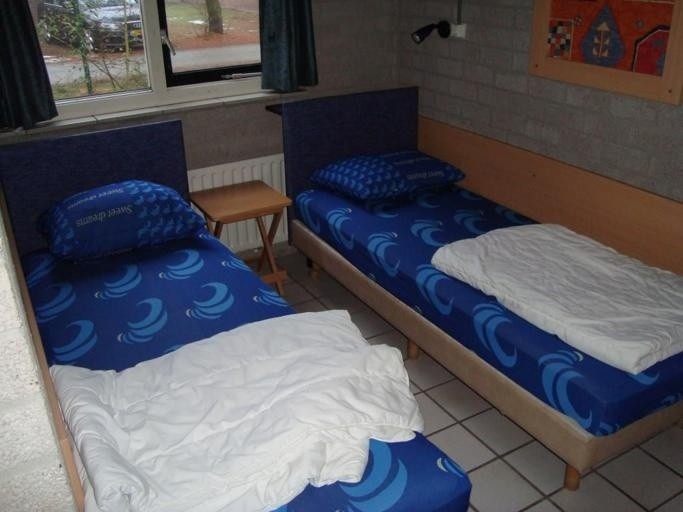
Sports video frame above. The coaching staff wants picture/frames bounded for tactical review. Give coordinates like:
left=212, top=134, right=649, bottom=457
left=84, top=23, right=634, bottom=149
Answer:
left=522, top=0, right=683, bottom=107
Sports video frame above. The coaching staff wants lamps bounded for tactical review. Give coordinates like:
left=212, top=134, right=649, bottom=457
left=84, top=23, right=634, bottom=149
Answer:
left=408, top=17, right=452, bottom=45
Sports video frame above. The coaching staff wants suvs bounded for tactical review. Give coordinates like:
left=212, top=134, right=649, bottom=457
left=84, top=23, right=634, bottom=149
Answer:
left=36, top=0, right=142, bottom=53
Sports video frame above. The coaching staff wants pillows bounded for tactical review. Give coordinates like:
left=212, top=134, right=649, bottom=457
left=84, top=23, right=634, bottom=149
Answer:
left=306, top=147, right=468, bottom=204
left=37, top=178, right=209, bottom=268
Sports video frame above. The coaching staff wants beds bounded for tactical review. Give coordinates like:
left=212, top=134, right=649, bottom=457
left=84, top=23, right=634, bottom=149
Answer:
left=274, top=83, right=682, bottom=493
left=0, top=118, right=477, bottom=512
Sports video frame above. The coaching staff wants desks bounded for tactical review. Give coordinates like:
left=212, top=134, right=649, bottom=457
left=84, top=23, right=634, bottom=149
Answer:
left=186, top=178, right=291, bottom=299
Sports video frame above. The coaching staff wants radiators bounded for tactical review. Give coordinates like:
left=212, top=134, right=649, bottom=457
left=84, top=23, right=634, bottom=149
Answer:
left=185, top=153, right=289, bottom=252
